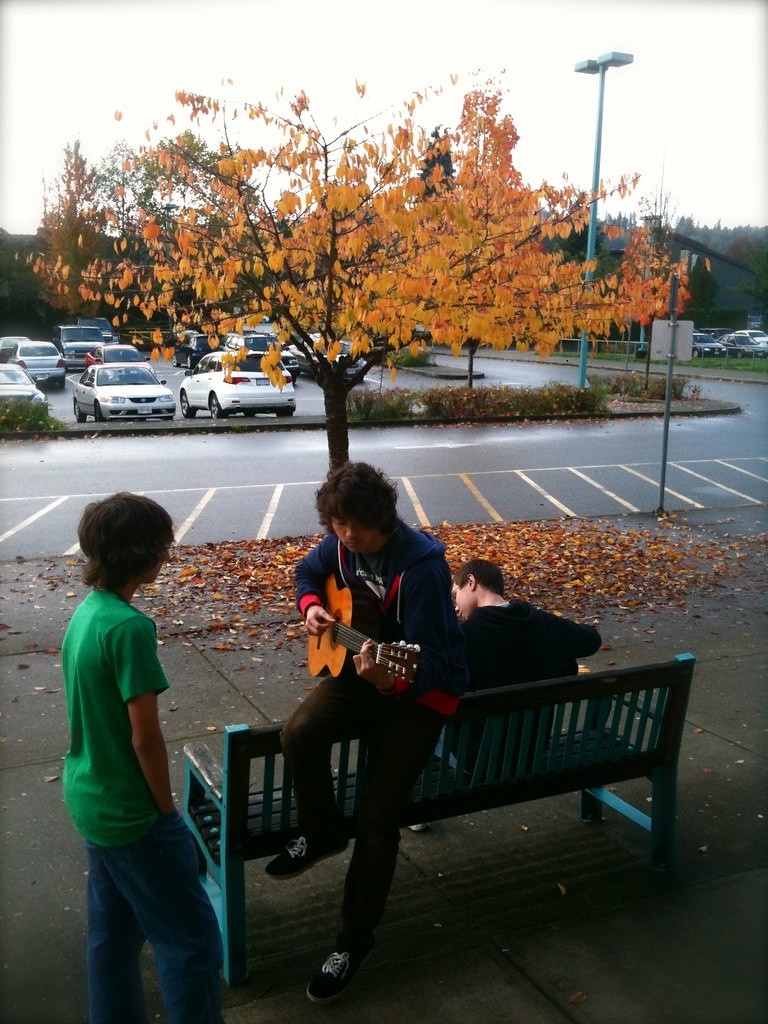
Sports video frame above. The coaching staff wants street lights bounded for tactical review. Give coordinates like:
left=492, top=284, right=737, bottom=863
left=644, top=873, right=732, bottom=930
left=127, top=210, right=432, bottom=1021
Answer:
left=639, top=214, right=664, bottom=352
left=575, top=51, right=634, bottom=389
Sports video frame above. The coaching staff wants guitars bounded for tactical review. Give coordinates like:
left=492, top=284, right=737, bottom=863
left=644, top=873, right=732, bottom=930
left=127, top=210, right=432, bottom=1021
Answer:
left=307, top=572, right=423, bottom=685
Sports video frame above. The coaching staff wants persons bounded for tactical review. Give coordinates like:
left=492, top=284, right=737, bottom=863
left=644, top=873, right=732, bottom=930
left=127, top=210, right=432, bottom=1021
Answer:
left=265, top=463, right=469, bottom=1003
left=407, top=562, right=602, bottom=831
left=62, top=493, right=225, bottom=1024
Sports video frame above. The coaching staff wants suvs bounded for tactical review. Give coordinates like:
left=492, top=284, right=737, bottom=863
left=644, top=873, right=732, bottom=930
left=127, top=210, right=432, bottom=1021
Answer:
left=218, top=332, right=299, bottom=386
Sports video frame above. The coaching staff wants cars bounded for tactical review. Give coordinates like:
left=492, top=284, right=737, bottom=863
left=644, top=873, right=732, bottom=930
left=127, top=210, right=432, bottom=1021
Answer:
left=716, top=333, right=766, bottom=360
left=74, top=317, right=119, bottom=347
left=0, top=337, right=33, bottom=364
left=179, top=351, right=297, bottom=418
left=0, top=364, right=48, bottom=417
left=72, top=365, right=176, bottom=421
left=732, top=330, right=768, bottom=354
left=50, top=325, right=113, bottom=373
left=85, top=345, right=156, bottom=378
left=693, top=333, right=727, bottom=358
left=172, top=333, right=221, bottom=369
left=6, top=341, right=67, bottom=388
left=287, top=333, right=369, bottom=382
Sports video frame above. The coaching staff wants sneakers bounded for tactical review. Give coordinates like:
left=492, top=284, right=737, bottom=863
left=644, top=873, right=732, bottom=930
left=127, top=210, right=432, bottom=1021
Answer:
left=306, top=945, right=376, bottom=1005
left=263, top=833, right=352, bottom=881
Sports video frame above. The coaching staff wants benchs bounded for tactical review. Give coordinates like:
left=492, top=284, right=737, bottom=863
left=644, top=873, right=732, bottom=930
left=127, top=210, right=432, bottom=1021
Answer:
left=181, top=653, right=697, bottom=989
